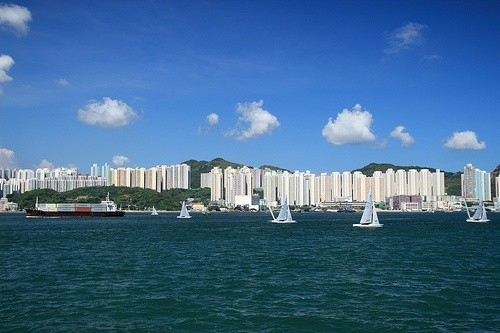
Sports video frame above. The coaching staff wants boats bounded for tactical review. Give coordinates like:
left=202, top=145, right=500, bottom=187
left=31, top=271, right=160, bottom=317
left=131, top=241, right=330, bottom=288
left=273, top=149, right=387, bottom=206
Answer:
left=25, top=192, right=125, bottom=217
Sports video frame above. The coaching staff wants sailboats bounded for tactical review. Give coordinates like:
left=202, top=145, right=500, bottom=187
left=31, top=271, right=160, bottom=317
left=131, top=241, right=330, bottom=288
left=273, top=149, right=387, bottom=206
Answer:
left=151, top=206, right=158, bottom=216
left=465, top=201, right=492, bottom=223
left=271, top=195, right=297, bottom=224
left=176, top=201, right=192, bottom=219
left=354, top=193, right=384, bottom=228
